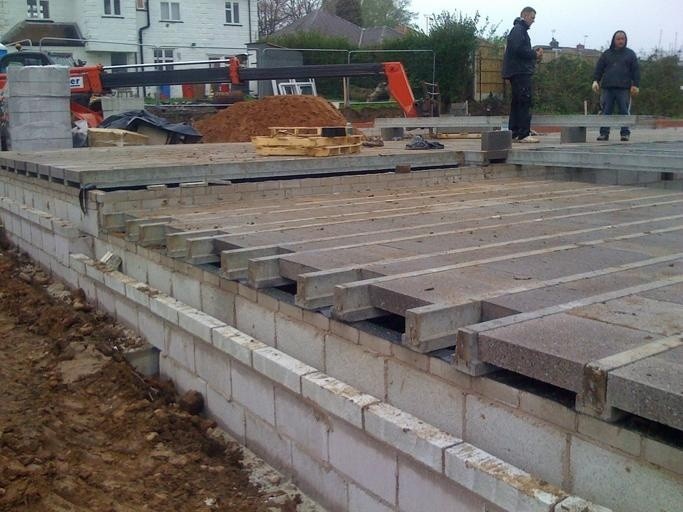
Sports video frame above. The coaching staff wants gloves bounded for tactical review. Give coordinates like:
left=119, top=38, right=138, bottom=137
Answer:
left=631, top=86, right=639, bottom=96
left=592, top=81, right=599, bottom=93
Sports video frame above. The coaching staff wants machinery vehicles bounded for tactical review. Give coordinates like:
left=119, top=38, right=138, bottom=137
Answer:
left=1, top=48, right=419, bottom=149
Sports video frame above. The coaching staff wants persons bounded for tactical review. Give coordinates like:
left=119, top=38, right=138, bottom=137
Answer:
left=501, top=7, right=544, bottom=142
left=592, top=30, right=640, bottom=141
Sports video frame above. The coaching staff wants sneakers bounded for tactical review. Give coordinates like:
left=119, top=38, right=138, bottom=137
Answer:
left=518, top=136, right=539, bottom=143
left=597, top=134, right=608, bottom=141
left=621, top=135, right=629, bottom=141
